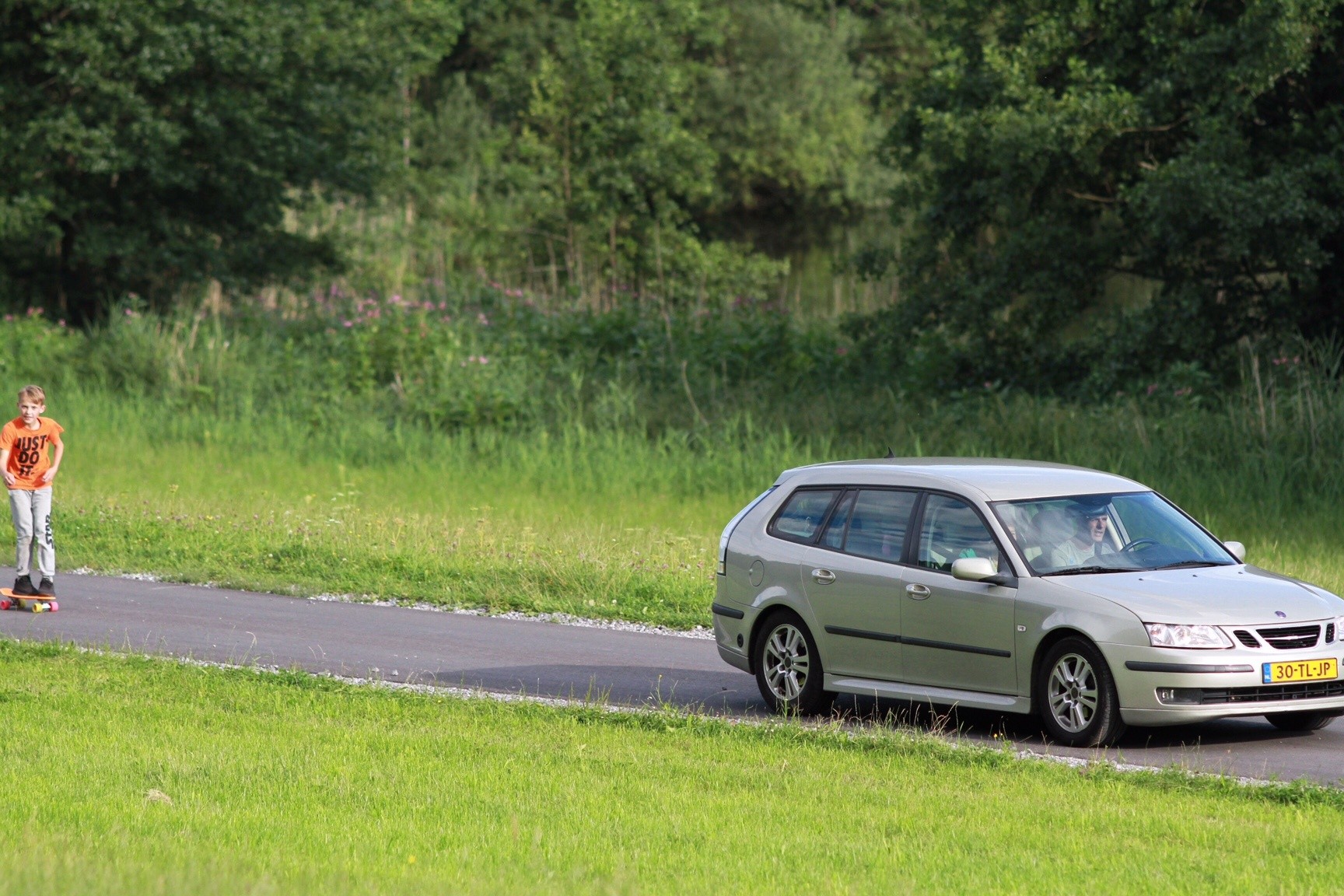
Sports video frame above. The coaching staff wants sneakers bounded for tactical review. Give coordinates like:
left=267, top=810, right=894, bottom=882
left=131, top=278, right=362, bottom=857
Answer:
left=37, top=579, right=55, bottom=596
left=12, top=576, right=38, bottom=595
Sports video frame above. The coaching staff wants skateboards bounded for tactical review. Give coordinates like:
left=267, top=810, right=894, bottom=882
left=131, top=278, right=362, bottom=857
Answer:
left=0, top=588, right=58, bottom=612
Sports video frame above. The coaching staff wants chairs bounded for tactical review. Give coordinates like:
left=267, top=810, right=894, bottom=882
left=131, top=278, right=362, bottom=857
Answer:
left=1020, top=509, right=1077, bottom=569
left=928, top=513, right=979, bottom=573
left=833, top=506, right=885, bottom=559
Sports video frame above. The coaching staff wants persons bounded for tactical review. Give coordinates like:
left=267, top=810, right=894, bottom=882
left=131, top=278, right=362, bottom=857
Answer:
left=959, top=520, right=1021, bottom=570
left=1051, top=506, right=1115, bottom=567
left=0, top=385, right=65, bottom=596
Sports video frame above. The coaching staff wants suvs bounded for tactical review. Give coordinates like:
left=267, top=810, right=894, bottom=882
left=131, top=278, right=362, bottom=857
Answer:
left=711, top=456, right=1344, bottom=749
